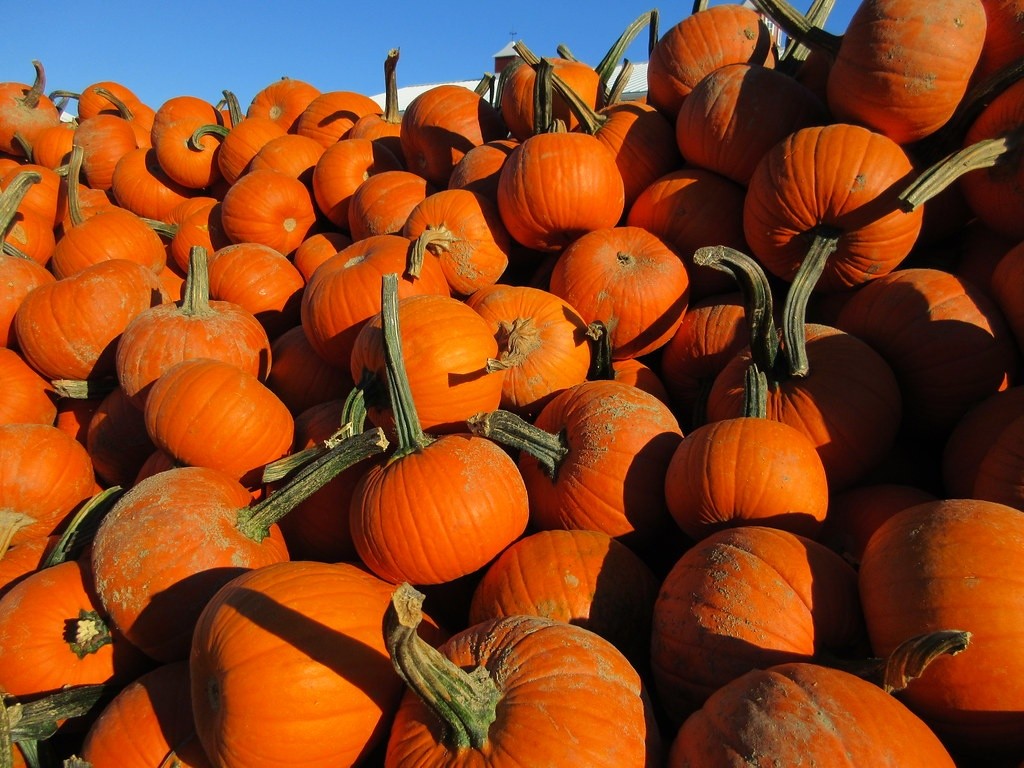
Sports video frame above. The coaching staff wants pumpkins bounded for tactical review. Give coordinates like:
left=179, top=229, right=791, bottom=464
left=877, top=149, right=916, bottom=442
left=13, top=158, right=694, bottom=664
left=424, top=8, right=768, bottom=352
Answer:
left=0, top=0, right=1024, bottom=767
